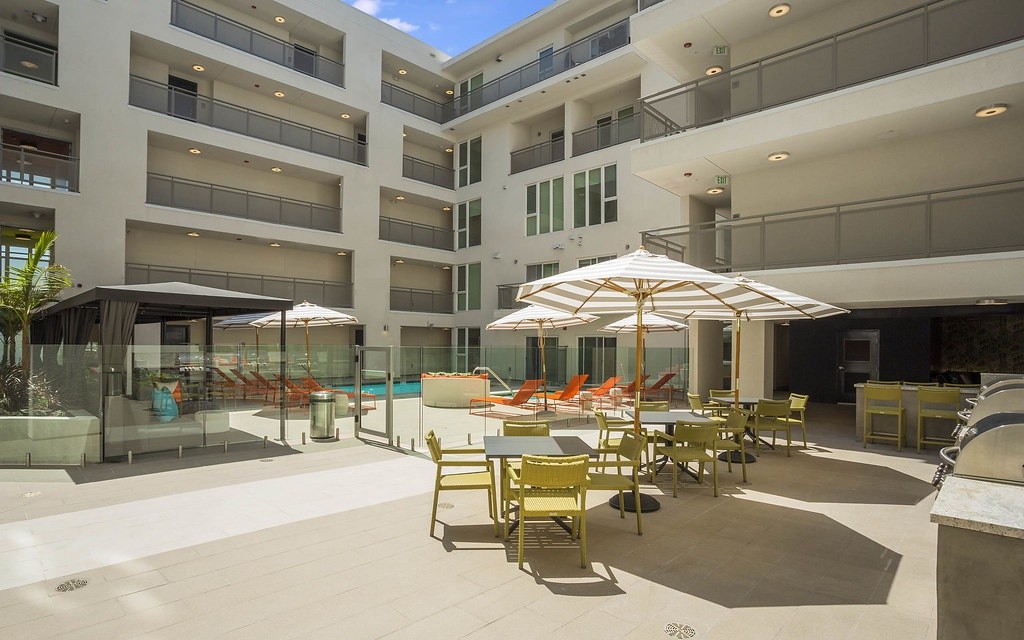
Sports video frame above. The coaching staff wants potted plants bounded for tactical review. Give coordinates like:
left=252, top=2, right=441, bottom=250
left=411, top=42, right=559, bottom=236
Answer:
left=134, top=368, right=156, bottom=400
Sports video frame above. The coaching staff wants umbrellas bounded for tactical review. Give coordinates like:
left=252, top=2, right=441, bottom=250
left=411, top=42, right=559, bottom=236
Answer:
left=212, top=300, right=359, bottom=387
left=515, top=246, right=784, bottom=469
left=486, top=304, right=601, bottom=410
left=597, top=312, right=690, bottom=401
left=649, top=272, right=851, bottom=451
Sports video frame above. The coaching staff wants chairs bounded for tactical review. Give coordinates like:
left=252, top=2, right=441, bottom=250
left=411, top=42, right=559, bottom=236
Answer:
left=593, top=376, right=622, bottom=397
left=699, top=407, right=749, bottom=484
left=903, top=382, right=939, bottom=387
left=747, top=398, right=792, bottom=458
left=863, top=384, right=905, bottom=455
left=424, top=428, right=499, bottom=539
left=916, top=385, right=962, bottom=455
left=572, top=428, right=645, bottom=538
left=615, top=372, right=675, bottom=402
left=653, top=420, right=721, bottom=497
left=531, top=374, right=588, bottom=404
left=944, top=383, right=981, bottom=388
left=502, top=418, right=550, bottom=518
left=639, top=400, right=670, bottom=481
left=768, top=393, right=809, bottom=449
left=594, top=407, right=651, bottom=482
left=866, top=380, right=901, bottom=385
left=587, top=373, right=650, bottom=406
left=468, top=379, right=557, bottom=415
left=710, top=389, right=736, bottom=416
left=687, top=392, right=729, bottom=440
left=151, top=365, right=377, bottom=414
left=504, top=453, right=589, bottom=570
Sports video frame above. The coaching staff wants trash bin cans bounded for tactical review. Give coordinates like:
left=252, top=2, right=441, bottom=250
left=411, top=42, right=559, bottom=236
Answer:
left=309, top=391, right=336, bottom=440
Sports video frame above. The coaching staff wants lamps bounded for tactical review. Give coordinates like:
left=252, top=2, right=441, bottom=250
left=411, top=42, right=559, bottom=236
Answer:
left=551, top=233, right=585, bottom=249
left=495, top=53, right=504, bottom=62
left=383, top=324, right=390, bottom=334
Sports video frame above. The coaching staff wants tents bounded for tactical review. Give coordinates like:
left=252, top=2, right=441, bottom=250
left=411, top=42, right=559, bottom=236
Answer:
left=30, top=281, right=293, bottom=461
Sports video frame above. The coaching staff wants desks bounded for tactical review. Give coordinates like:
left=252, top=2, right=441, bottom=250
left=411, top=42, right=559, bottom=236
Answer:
left=853, top=383, right=981, bottom=450
left=625, top=411, right=710, bottom=484
left=708, top=396, right=771, bottom=463
left=483, top=435, right=599, bottom=543
